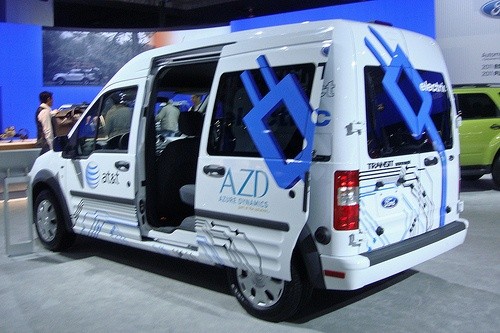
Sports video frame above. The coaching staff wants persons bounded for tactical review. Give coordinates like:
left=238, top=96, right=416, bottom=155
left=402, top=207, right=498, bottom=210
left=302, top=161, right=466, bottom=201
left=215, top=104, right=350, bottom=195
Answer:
left=155, top=100, right=181, bottom=134
left=106, top=94, right=126, bottom=119
left=35, top=92, right=61, bottom=155
left=103, top=95, right=135, bottom=141
left=187, top=94, right=203, bottom=112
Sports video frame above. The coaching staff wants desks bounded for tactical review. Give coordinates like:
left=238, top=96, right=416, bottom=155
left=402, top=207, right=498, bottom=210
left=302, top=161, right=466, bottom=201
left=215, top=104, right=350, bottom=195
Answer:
left=0, top=139, right=42, bottom=152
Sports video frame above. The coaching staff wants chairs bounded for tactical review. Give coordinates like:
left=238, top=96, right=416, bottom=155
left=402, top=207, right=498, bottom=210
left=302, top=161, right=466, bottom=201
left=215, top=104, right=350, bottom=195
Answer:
left=156, top=111, right=216, bottom=213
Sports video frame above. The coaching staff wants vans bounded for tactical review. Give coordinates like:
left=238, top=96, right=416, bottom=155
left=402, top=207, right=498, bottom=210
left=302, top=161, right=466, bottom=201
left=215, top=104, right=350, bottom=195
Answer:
left=26, top=20, right=469, bottom=322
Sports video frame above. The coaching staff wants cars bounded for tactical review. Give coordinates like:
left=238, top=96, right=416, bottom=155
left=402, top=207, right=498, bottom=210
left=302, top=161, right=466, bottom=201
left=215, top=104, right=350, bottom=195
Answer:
left=449, top=83, right=500, bottom=188
